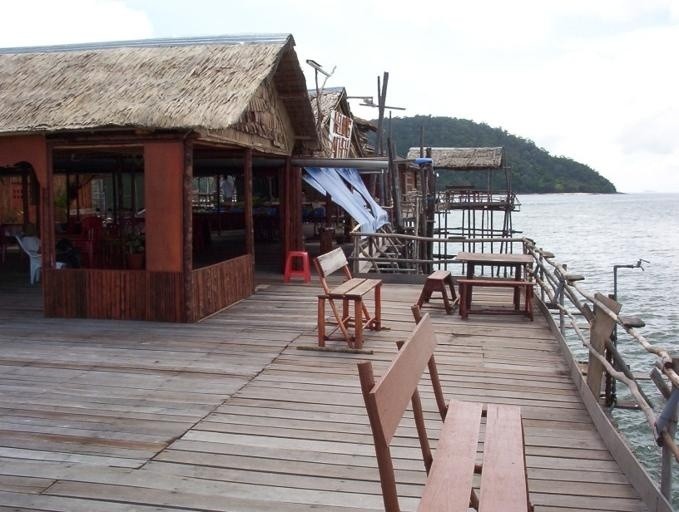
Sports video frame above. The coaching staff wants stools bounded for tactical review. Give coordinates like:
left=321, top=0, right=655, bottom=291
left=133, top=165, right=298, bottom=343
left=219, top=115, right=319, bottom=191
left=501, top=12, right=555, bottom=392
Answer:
left=284, top=251, right=311, bottom=283
left=197, top=193, right=216, bottom=211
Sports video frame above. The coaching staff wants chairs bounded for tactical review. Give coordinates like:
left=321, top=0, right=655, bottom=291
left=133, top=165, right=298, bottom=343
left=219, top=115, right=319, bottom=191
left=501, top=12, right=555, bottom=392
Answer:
left=2, top=224, right=25, bottom=268
left=16, top=235, right=65, bottom=285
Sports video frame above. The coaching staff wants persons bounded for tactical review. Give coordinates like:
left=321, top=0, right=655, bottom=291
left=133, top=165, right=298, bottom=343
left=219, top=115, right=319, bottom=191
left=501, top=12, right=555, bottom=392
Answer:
left=219, top=174, right=234, bottom=211
left=97, top=207, right=112, bottom=220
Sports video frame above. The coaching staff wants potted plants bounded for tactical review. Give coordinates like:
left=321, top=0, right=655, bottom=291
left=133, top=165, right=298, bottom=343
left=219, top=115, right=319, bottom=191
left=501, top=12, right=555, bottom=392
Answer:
left=124, top=230, right=145, bottom=270
left=77, top=215, right=106, bottom=269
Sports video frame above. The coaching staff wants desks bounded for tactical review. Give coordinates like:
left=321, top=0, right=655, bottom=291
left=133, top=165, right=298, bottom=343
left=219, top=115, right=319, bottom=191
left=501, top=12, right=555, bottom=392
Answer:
left=455, top=252, right=534, bottom=311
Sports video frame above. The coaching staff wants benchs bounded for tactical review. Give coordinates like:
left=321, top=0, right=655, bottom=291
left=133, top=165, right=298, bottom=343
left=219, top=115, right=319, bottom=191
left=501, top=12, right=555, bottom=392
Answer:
left=457, top=279, right=537, bottom=322
left=417, top=271, right=459, bottom=314
left=357, top=305, right=533, bottom=512
left=313, top=246, right=383, bottom=348
